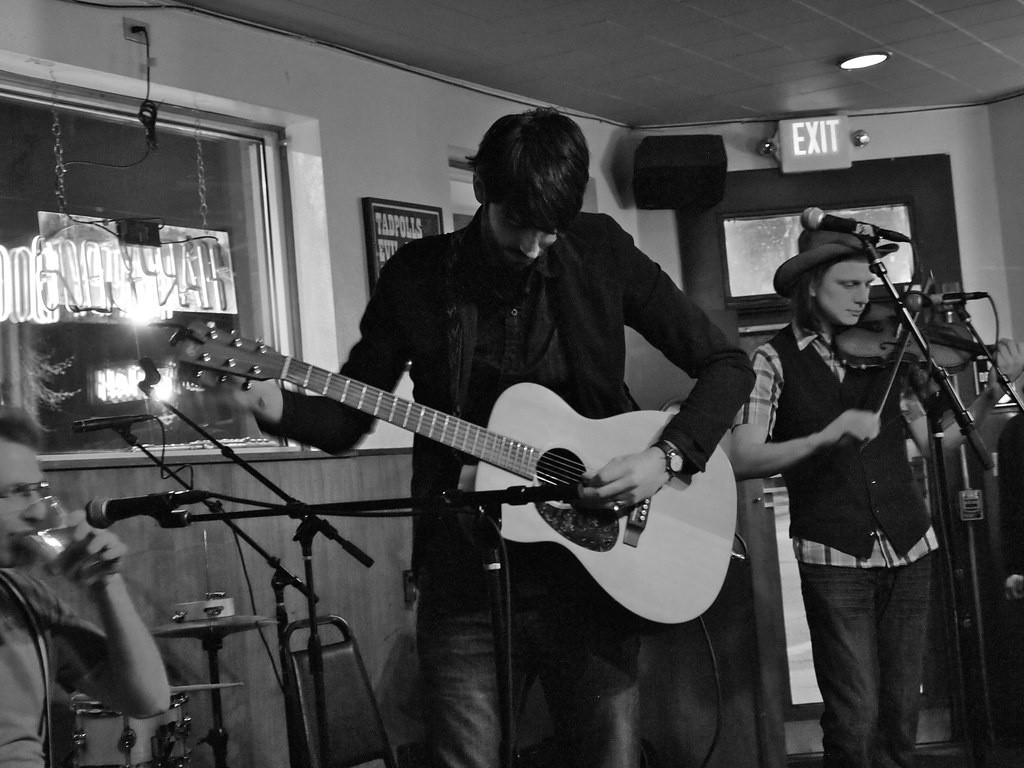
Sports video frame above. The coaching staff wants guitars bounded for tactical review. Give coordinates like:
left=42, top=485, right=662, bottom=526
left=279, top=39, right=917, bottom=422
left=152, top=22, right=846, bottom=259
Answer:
left=154, top=312, right=742, bottom=631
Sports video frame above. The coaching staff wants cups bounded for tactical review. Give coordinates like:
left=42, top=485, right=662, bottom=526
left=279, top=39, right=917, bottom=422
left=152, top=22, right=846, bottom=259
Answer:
left=12, top=495, right=117, bottom=584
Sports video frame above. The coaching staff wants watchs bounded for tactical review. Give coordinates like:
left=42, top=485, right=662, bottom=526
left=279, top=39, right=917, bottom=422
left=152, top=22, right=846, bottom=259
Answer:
left=650, top=441, right=685, bottom=478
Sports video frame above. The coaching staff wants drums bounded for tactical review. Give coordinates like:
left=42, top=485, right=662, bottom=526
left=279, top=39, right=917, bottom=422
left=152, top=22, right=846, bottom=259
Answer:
left=69, top=691, right=189, bottom=767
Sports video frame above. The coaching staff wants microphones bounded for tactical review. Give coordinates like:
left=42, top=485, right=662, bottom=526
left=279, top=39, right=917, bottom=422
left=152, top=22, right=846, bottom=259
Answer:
left=86, top=490, right=212, bottom=528
left=907, top=291, right=988, bottom=311
left=800, top=207, right=911, bottom=244
left=72, top=415, right=155, bottom=431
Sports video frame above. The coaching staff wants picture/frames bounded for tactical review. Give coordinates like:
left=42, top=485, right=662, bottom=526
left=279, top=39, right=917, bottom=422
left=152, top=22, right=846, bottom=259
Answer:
left=361, top=196, right=444, bottom=370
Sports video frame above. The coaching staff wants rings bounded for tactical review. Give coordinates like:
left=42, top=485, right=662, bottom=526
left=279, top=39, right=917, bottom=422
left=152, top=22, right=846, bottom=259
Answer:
left=613, top=501, right=620, bottom=513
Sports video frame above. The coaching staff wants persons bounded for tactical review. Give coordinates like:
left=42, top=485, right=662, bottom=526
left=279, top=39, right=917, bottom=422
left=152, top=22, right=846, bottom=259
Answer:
left=0, top=404, right=171, bottom=768
left=729, top=227, right=1024, bottom=768
left=197, top=101, right=757, bottom=768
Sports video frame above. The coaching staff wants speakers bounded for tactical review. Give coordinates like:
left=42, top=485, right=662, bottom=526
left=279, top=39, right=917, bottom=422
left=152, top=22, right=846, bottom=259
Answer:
left=632, top=133, right=728, bottom=208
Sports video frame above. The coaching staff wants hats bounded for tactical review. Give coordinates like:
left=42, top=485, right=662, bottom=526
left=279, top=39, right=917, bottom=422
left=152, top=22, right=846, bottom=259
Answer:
left=773, top=229, right=899, bottom=298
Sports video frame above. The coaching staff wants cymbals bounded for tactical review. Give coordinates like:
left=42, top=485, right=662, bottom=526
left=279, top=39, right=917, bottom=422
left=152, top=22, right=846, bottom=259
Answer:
left=148, top=615, right=275, bottom=638
left=169, top=680, right=245, bottom=695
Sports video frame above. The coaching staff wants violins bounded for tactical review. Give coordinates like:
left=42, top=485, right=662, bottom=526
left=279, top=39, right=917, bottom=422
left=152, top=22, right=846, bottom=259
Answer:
left=832, top=300, right=1024, bottom=376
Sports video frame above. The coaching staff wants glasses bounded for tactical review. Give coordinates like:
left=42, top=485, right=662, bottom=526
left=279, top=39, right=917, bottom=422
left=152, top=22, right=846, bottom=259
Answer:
left=0, top=480, right=50, bottom=502
